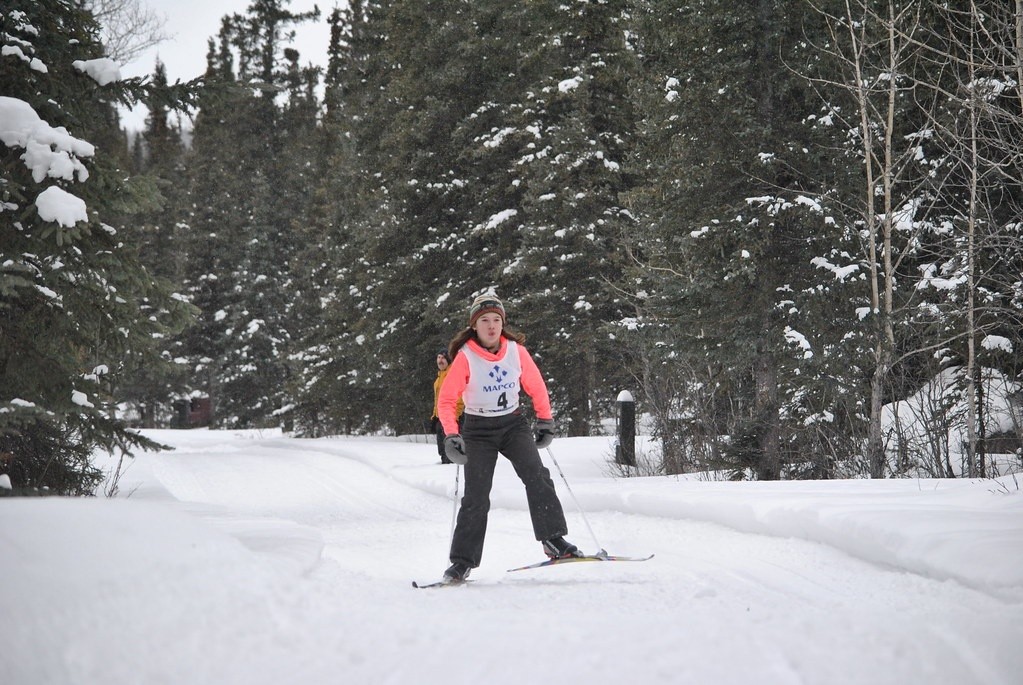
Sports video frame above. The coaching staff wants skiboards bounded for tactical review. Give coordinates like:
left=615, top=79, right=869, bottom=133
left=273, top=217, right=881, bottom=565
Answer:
left=411, top=552, right=657, bottom=589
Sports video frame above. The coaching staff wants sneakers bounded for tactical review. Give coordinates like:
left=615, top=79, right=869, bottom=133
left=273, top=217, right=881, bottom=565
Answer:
left=443, top=563, right=471, bottom=582
left=542, top=536, right=577, bottom=559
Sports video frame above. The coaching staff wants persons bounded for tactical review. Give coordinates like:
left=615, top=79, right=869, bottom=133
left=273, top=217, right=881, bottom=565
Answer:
left=435, top=294, right=577, bottom=580
left=430, top=348, right=465, bottom=464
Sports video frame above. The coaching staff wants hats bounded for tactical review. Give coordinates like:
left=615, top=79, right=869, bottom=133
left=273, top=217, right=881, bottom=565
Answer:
left=438, top=348, right=451, bottom=365
left=469, top=294, right=505, bottom=327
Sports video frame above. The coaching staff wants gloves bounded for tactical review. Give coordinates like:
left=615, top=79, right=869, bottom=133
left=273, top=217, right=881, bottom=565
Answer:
left=444, top=434, right=468, bottom=464
left=534, top=418, right=555, bottom=449
